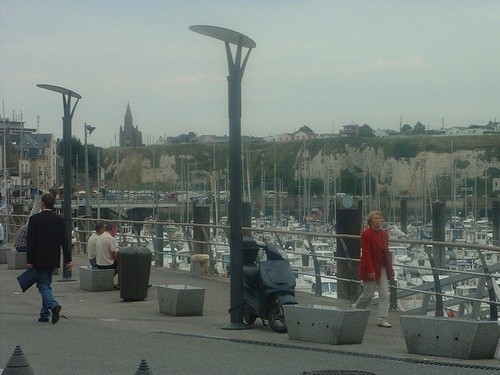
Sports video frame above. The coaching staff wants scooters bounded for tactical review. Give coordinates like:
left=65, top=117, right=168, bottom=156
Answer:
left=226, top=236, right=299, bottom=333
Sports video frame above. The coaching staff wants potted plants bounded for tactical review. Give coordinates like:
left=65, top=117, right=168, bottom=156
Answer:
left=12, top=190, right=25, bottom=214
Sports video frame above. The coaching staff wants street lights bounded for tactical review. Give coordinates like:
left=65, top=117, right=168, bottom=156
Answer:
left=190, top=24, right=256, bottom=330
left=36, top=83, right=83, bottom=282
left=85, top=123, right=97, bottom=243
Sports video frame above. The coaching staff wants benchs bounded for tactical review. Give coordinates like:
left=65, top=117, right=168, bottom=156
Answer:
left=157, top=284, right=205, bottom=316
left=400, top=314, right=500, bottom=360
left=6, top=251, right=27, bottom=269
left=0, top=246, right=11, bottom=264
left=283, top=304, right=371, bottom=345
left=79, top=265, right=115, bottom=292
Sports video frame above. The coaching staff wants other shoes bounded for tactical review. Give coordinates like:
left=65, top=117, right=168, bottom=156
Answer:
left=38, top=317, right=49, bottom=322
left=52, top=304, right=61, bottom=323
left=378, top=321, right=392, bottom=326
left=113, top=283, right=120, bottom=290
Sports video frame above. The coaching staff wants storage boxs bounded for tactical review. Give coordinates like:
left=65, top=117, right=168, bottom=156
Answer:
left=243, top=237, right=260, bottom=264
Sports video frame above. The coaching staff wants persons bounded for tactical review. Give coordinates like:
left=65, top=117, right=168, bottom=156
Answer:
left=95, top=222, right=121, bottom=276
left=15, top=224, right=27, bottom=252
left=0, top=223, right=4, bottom=244
left=354, top=211, right=391, bottom=328
left=27, top=193, right=72, bottom=324
left=88, top=221, right=107, bottom=269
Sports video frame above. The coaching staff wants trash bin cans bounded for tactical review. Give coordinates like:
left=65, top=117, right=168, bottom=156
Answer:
left=117, top=246, right=152, bottom=302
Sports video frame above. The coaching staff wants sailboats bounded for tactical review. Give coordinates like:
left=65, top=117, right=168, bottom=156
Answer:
left=0, top=96, right=500, bottom=318
